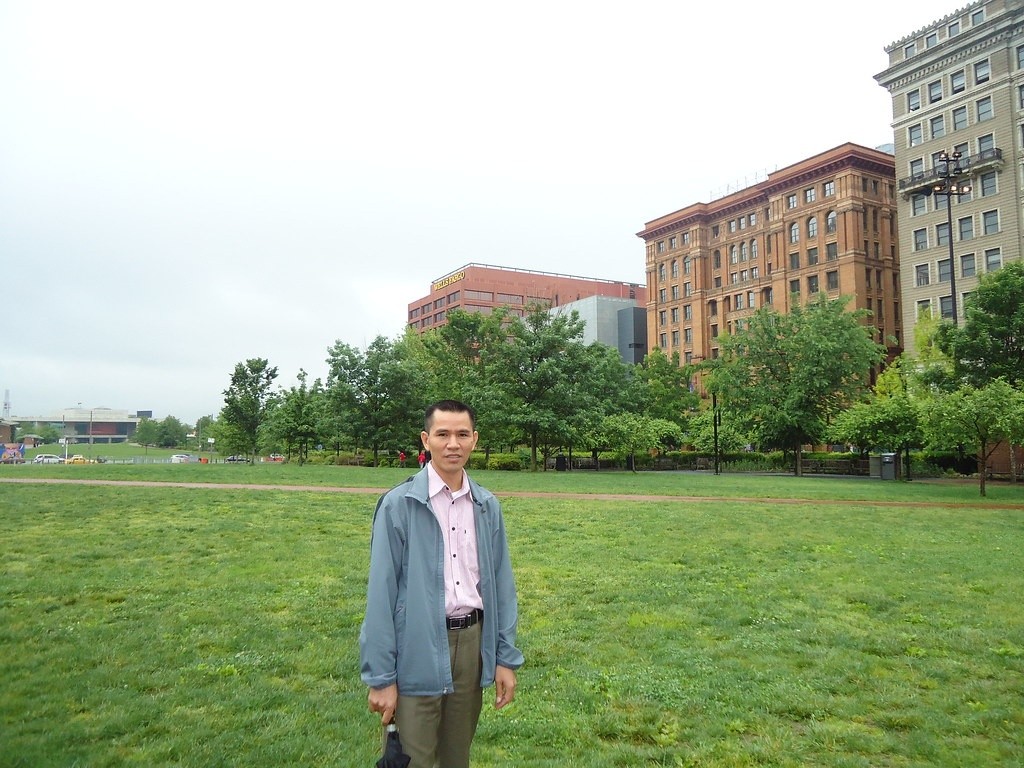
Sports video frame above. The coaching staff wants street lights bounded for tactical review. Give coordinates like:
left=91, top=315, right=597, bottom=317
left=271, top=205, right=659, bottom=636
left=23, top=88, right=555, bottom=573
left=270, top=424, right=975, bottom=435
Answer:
left=932, top=152, right=973, bottom=333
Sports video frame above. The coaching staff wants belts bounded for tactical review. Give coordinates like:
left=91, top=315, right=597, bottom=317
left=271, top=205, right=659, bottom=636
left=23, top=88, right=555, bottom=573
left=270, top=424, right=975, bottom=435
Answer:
left=446, top=609, right=483, bottom=630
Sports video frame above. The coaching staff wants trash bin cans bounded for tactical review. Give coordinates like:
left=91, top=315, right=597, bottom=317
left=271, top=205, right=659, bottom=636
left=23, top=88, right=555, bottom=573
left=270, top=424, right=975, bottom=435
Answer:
left=880, top=452, right=898, bottom=480
left=555, top=454, right=567, bottom=471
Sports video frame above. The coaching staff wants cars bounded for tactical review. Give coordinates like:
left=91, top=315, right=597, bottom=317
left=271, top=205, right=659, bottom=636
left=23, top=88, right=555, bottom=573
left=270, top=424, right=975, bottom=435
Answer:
left=67, top=455, right=98, bottom=464
left=34, top=454, right=64, bottom=464
left=226, top=454, right=250, bottom=464
left=171, top=455, right=188, bottom=462
left=261, top=454, right=285, bottom=461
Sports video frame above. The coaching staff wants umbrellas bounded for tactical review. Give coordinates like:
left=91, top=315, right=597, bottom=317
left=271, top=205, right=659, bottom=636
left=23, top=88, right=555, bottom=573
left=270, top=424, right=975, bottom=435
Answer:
left=375, top=708, right=412, bottom=768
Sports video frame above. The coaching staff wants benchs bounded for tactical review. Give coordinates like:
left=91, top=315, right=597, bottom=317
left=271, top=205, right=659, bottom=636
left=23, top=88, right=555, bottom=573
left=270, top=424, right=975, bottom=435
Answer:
left=348, top=454, right=365, bottom=466
left=786, top=458, right=870, bottom=476
left=689, top=458, right=712, bottom=471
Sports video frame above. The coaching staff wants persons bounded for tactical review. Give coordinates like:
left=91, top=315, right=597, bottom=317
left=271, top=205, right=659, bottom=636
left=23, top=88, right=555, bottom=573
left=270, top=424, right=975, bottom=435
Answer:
left=0, top=444, right=6, bottom=459
left=16, top=440, right=25, bottom=458
left=399, top=452, right=405, bottom=468
left=418, top=451, right=425, bottom=470
left=358, top=400, right=526, bottom=768
left=317, top=444, right=322, bottom=451
left=34, top=439, right=38, bottom=448
